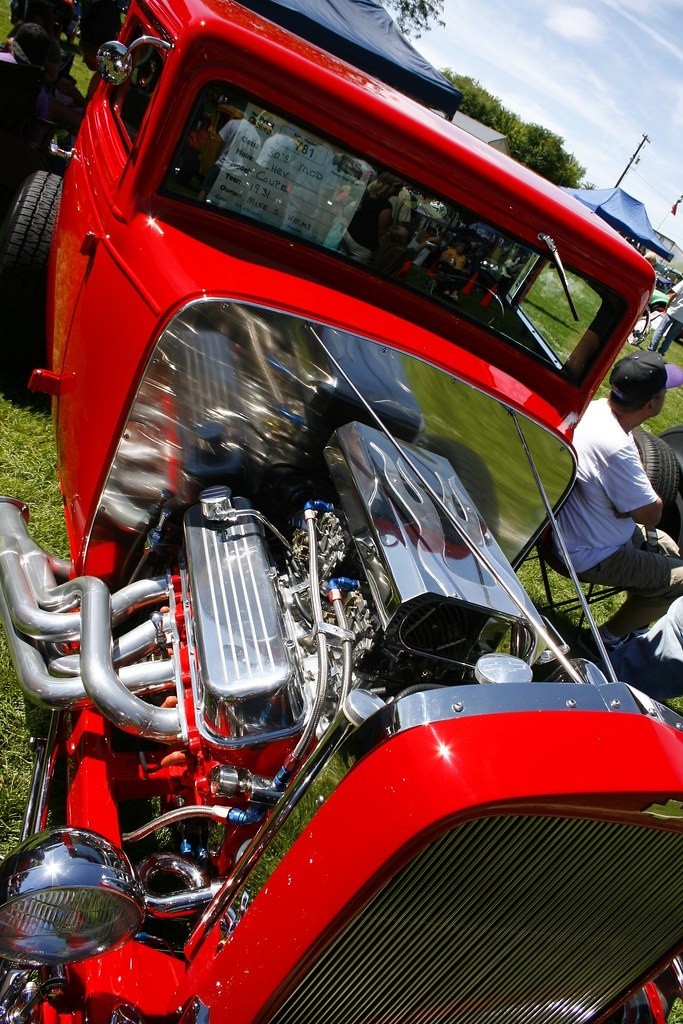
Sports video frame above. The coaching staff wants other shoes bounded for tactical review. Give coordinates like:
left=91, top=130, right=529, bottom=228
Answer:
left=448, top=295, right=460, bottom=304
left=634, top=623, right=649, bottom=633
left=442, top=290, right=449, bottom=297
left=578, top=627, right=630, bottom=662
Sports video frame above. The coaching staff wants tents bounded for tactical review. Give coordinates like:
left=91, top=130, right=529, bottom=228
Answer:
left=239, top=0, right=465, bottom=124
left=559, top=186, right=675, bottom=264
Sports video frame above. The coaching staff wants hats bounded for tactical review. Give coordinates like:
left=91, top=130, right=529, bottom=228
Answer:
left=644, top=252, right=657, bottom=266
left=609, top=351, right=683, bottom=401
left=12, top=23, right=49, bottom=65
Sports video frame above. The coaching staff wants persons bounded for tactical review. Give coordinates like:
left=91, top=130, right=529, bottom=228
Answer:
left=211, top=108, right=526, bottom=309
left=538, top=347, right=683, bottom=700
left=648, top=281, right=683, bottom=355
left=0, top=0, right=124, bottom=170
left=617, top=230, right=657, bottom=267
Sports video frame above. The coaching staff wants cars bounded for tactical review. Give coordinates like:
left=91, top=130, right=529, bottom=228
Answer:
left=0, top=0, right=683, bottom=1024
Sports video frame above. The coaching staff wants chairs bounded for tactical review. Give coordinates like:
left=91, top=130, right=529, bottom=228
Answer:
left=518, top=521, right=627, bottom=636
